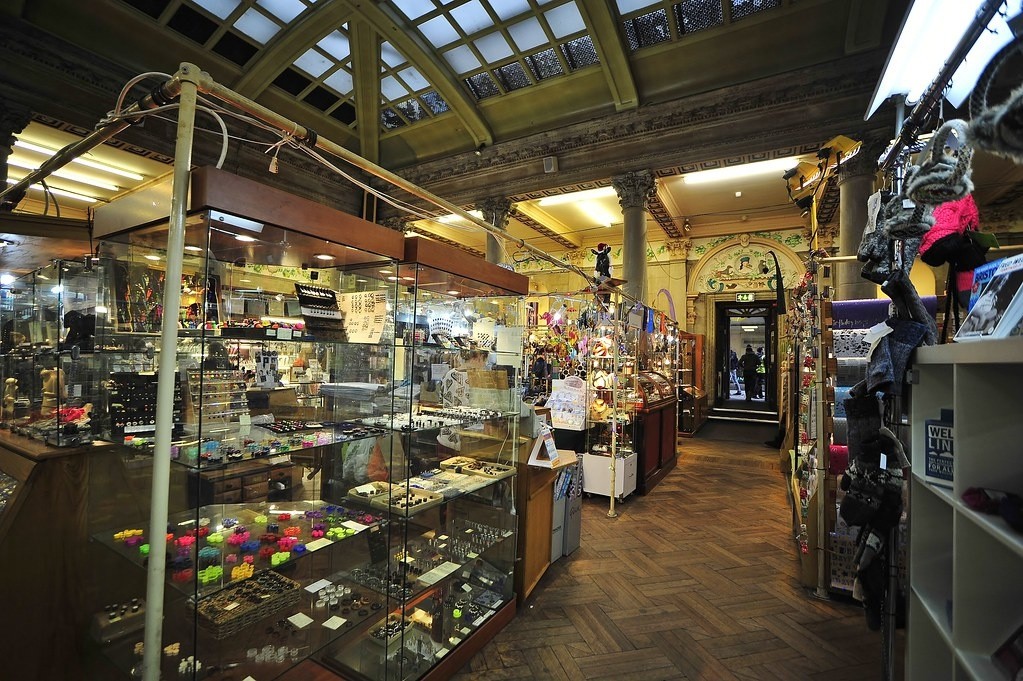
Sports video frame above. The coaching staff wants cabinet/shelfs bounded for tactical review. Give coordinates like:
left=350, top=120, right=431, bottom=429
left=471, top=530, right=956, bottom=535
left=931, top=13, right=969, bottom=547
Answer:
left=904, top=336, right=1023, bottom=681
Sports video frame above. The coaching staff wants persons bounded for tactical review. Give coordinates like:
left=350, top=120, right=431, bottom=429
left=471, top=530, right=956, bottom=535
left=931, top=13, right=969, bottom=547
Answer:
left=754, top=347, right=766, bottom=399
left=738, top=347, right=761, bottom=400
left=437, top=348, right=489, bottom=469
left=730, top=350, right=742, bottom=395
left=204, top=341, right=237, bottom=370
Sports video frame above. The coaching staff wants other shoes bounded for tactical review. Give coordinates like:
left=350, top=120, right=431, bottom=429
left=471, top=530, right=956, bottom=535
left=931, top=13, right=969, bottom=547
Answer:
left=733, top=391, right=765, bottom=402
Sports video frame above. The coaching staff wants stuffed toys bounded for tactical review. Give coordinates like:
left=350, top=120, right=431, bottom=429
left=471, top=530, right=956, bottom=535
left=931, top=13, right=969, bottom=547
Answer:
left=591, top=242, right=614, bottom=278
